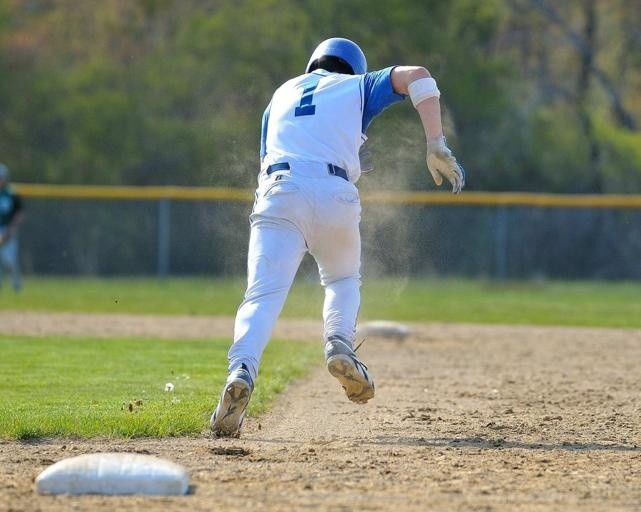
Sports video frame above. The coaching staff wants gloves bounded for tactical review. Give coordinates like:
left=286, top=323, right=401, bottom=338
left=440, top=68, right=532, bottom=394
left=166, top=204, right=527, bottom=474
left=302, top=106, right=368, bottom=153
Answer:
left=426, top=136, right=465, bottom=194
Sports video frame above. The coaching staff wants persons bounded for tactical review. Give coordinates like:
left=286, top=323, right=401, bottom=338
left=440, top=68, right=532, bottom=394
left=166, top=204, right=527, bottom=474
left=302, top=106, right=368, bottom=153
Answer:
left=212, top=38, right=464, bottom=442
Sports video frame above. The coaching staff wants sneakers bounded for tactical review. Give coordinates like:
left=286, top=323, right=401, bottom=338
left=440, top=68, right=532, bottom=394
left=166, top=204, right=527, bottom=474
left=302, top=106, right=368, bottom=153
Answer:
left=324, top=335, right=375, bottom=404
left=210, top=363, right=254, bottom=439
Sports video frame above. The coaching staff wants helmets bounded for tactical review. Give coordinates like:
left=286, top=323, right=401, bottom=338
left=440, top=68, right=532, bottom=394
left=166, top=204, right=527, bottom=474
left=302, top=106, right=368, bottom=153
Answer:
left=305, top=37, right=367, bottom=76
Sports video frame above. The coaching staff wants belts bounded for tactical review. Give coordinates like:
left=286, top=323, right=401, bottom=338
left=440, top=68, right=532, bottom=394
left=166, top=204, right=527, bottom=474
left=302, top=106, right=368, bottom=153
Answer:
left=267, top=162, right=348, bottom=181
